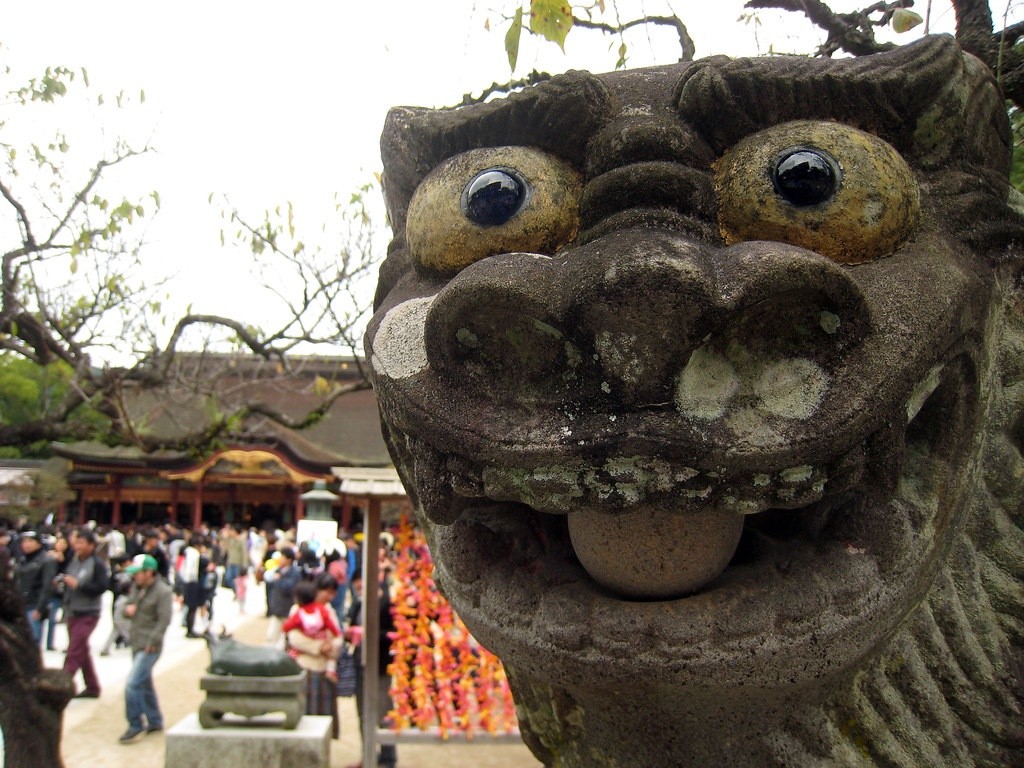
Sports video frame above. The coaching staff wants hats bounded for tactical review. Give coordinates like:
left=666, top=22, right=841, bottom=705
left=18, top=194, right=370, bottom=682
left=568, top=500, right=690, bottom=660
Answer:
left=123, top=554, right=157, bottom=573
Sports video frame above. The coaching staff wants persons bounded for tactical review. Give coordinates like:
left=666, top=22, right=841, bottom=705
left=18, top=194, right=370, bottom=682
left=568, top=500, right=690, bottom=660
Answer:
left=119, top=552, right=171, bottom=744
left=52, top=531, right=108, bottom=700
left=0, top=517, right=398, bottom=768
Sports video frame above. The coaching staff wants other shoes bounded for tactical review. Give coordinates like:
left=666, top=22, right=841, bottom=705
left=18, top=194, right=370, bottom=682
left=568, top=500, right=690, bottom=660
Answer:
left=145, top=723, right=162, bottom=733
left=76, top=689, right=98, bottom=698
left=120, top=725, right=147, bottom=742
left=186, top=631, right=201, bottom=639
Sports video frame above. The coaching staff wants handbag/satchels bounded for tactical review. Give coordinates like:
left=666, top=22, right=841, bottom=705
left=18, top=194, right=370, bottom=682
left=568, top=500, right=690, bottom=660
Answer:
left=327, top=641, right=360, bottom=696
